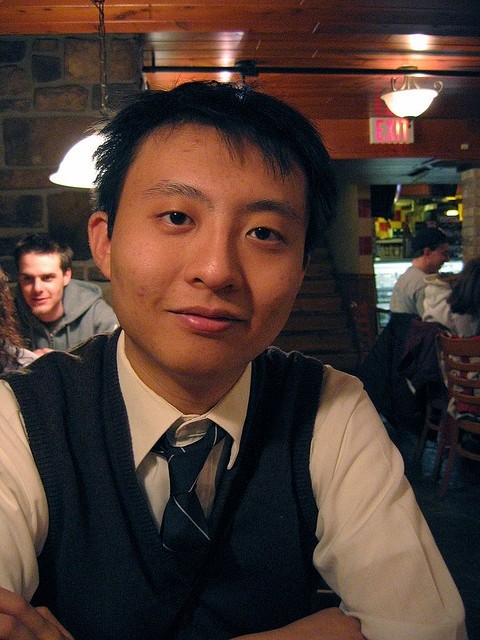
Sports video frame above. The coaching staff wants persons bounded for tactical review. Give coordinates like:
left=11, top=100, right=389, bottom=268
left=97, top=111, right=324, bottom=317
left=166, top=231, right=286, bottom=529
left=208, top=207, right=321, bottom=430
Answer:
left=12, top=229, right=118, bottom=349
left=446, top=255, right=480, bottom=338
left=390, top=227, right=456, bottom=338
left=0, top=81, right=469, bottom=640
left=0, top=272, right=38, bottom=381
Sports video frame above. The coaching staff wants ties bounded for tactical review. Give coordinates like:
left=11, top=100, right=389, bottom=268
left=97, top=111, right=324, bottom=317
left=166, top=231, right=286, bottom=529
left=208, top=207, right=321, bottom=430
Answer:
left=144, top=423, right=226, bottom=578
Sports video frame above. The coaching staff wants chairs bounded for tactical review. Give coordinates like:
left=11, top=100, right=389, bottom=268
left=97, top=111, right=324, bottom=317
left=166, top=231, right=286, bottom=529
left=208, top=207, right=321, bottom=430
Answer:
left=390, top=311, right=445, bottom=460
left=438, top=335, right=479, bottom=497
left=391, top=370, right=396, bottom=373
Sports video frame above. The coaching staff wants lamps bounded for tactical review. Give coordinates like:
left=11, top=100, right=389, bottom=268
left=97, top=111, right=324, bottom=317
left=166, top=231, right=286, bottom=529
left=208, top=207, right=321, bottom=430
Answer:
left=49, top=0, right=133, bottom=191
left=381, top=79, right=444, bottom=132
